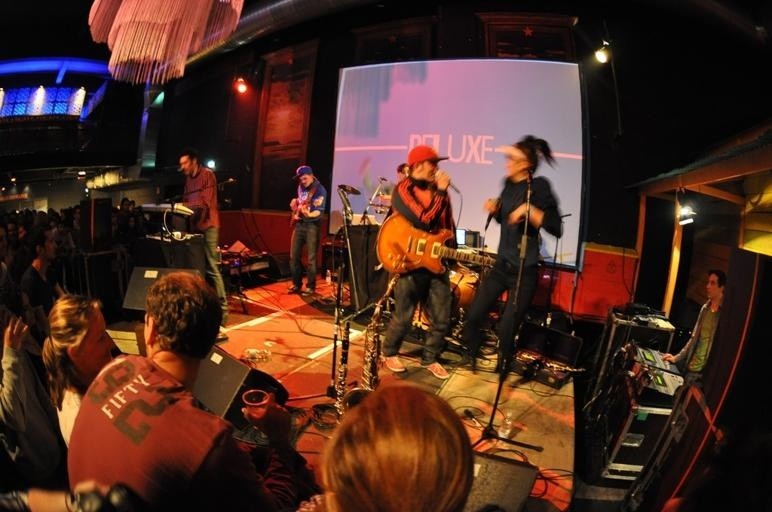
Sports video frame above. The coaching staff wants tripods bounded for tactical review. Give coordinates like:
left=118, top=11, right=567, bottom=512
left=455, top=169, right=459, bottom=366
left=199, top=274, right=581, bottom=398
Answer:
left=464, top=215, right=545, bottom=454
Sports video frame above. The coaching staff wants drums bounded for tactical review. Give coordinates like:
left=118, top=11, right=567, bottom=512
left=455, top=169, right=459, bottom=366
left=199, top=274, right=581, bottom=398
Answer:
left=447, top=268, right=479, bottom=310
left=411, top=300, right=431, bottom=331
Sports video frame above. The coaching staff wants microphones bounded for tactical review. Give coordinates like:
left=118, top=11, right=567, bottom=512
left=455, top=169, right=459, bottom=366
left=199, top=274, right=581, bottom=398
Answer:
left=435, top=168, right=461, bottom=197
left=337, top=187, right=354, bottom=215
left=485, top=196, right=502, bottom=231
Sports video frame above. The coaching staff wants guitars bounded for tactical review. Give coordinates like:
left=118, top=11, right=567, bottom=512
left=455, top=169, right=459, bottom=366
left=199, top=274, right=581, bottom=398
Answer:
left=290, top=200, right=311, bottom=227
left=376, top=212, right=497, bottom=274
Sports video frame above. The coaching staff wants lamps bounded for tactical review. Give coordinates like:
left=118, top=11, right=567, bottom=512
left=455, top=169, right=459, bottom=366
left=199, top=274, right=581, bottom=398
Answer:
left=87, top=0, right=245, bottom=87
left=676, top=183, right=700, bottom=227
left=595, top=35, right=616, bottom=64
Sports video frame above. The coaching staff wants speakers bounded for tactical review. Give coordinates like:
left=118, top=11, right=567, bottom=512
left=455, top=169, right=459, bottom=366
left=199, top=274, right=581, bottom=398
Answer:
left=461, top=449, right=539, bottom=512
left=187, top=342, right=289, bottom=433
left=122, top=266, right=205, bottom=322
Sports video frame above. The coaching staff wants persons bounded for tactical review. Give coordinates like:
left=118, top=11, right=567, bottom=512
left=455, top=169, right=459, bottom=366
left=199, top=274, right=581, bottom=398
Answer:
left=664, top=270, right=725, bottom=390
left=1, top=198, right=148, bottom=397
left=295, top=384, right=473, bottom=511
left=2, top=479, right=115, bottom=511
left=464, top=135, right=563, bottom=403
left=68, top=271, right=308, bottom=511
left=175, top=153, right=229, bottom=323
left=385, top=162, right=410, bottom=219
left=44, top=295, right=116, bottom=450
left=1, top=304, right=67, bottom=488
left=383, top=147, right=457, bottom=380
left=289, top=165, right=326, bottom=296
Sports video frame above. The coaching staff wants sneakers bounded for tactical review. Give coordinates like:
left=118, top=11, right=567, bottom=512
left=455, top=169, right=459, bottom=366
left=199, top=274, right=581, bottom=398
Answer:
left=385, top=356, right=405, bottom=372
left=427, top=361, right=450, bottom=380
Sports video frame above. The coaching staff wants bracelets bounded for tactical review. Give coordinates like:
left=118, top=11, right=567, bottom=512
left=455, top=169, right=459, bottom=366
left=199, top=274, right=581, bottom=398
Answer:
left=63, top=489, right=79, bottom=511
left=528, top=204, right=535, bottom=220
left=677, top=348, right=686, bottom=361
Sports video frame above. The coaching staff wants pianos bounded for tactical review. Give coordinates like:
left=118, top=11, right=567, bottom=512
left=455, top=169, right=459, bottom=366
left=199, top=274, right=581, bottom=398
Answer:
left=139, top=203, right=195, bottom=216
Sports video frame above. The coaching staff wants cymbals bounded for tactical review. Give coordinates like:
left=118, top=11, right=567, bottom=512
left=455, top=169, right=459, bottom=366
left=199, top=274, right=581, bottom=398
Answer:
left=368, top=203, right=390, bottom=214
left=377, top=194, right=392, bottom=205
left=338, top=185, right=360, bottom=194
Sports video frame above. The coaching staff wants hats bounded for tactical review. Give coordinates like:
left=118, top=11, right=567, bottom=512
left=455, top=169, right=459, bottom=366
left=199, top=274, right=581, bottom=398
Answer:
left=407, top=146, right=449, bottom=167
left=293, top=166, right=312, bottom=179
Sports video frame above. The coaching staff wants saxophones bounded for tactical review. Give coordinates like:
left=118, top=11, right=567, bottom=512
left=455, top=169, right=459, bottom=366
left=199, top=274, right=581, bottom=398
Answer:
left=341, top=274, right=400, bottom=415
